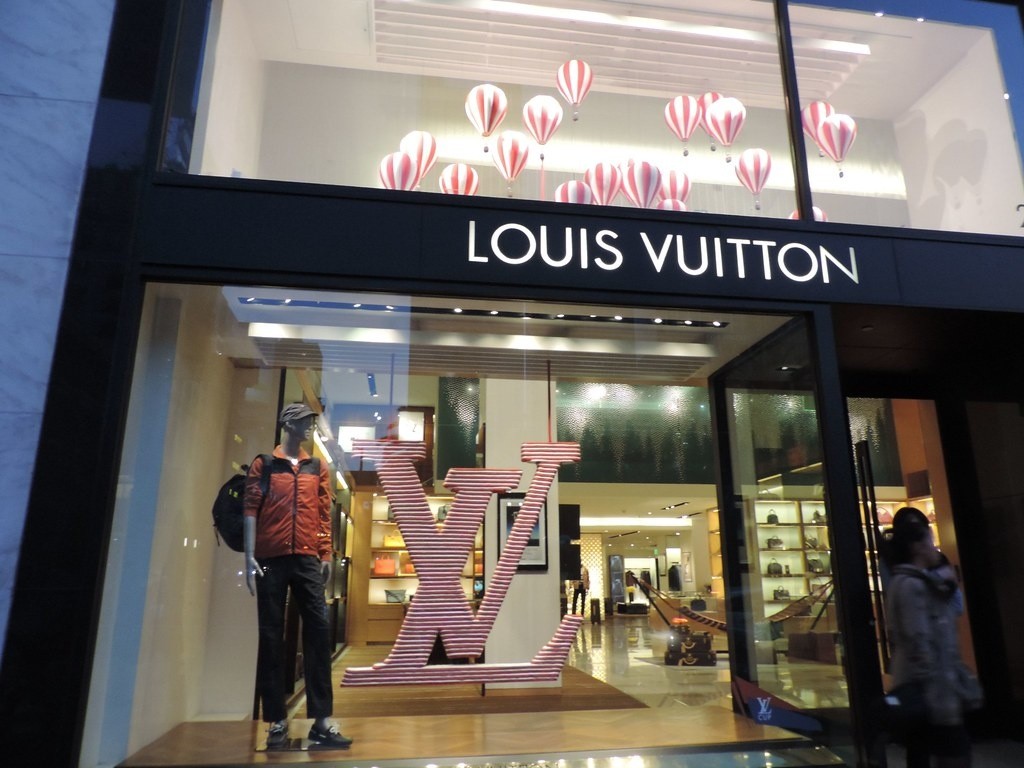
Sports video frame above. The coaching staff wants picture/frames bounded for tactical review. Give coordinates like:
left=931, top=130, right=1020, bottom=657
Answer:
left=498, top=493, right=549, bottom=571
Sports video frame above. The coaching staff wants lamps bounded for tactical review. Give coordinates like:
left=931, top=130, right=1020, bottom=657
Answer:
left=368, top=373, right=377, bottom=395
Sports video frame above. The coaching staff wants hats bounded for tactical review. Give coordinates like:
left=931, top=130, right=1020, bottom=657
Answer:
left=278, top=405, right=319, bottom=426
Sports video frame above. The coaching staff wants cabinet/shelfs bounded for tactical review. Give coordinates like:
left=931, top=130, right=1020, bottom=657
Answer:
left=782, top=603, right=843, bottom=664
left=347, top=492, right=484, bottom=645
left=705, top=501, right=939, bottom=605
left=296, top=426, right=350, bottom=668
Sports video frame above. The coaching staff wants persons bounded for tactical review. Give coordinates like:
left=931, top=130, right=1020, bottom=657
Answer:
left=244, top=403, right=351, bottom=746
left=893, top=507, right=962, bottom=623
left=878, top=523, right=968, bottom=768
left=569, top=564, right=590, bottom=618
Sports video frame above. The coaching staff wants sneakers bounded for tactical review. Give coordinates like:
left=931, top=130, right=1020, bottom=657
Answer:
left=265, top=721, right=288, bottom=749
left=308, top=723, right=353, bottom=747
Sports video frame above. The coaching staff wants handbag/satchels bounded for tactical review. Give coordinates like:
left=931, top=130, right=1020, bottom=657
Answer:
left=211, top=454, right=272, bottom=552
